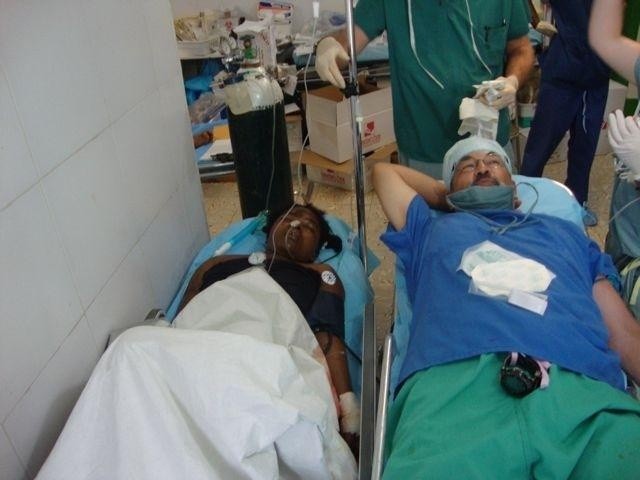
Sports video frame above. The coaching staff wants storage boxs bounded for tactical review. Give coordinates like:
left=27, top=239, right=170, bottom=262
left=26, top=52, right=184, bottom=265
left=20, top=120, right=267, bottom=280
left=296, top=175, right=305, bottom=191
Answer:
left=303, top=75, right=402, bottom=164
left=298, top=144, right=404, bottom=191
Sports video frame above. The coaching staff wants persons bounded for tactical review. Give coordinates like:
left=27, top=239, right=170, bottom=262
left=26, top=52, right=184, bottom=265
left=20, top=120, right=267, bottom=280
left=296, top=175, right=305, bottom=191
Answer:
left=588, top=0, right=640, bottom=327
left=31, top=204, right=361, bottom=480
left=372, top=136, right=640, bottom=480
left=520, top=0, right=610, bottom=227
left=316, top=0, right=535, bottom=185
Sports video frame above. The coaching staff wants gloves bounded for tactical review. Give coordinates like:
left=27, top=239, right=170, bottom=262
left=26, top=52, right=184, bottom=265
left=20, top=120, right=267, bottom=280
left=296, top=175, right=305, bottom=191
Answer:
left=314, top=37, right=350, bottom=89
left=606, top=109, right=640, bottom=175
left=475, top=75, right=519, bottom=110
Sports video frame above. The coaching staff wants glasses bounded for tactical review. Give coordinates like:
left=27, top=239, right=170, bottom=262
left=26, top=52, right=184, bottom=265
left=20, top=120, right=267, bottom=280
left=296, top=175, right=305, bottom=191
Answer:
left=452, top=153, right=512, bottom=189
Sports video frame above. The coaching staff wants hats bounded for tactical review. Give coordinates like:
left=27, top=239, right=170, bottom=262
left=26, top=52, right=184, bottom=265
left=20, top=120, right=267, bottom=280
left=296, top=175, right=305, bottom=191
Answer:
left=442, top=137, right=512, bottom=192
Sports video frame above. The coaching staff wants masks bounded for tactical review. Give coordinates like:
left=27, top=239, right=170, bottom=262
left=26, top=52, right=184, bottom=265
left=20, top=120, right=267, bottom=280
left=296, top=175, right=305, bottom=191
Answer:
left=445, top=187, right=515, bottom=213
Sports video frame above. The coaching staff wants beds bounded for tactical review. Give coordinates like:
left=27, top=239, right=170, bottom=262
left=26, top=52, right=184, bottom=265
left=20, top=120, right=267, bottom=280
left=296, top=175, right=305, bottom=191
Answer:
left=152, top=203, right=361, bottom=480
left=387, top=165, right=604, bottom=480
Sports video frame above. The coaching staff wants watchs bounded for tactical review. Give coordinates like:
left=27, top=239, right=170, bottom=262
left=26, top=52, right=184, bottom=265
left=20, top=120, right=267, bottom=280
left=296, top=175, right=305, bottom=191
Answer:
left=500, top=352, right=541, bottom=397
